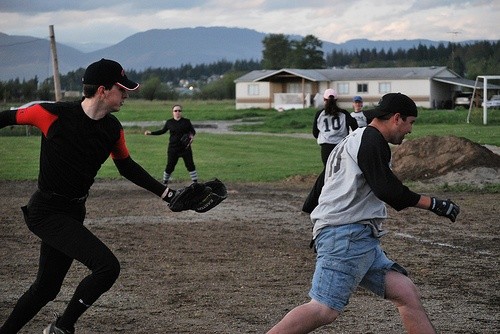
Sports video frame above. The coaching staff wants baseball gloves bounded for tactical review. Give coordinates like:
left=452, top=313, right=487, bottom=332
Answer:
left=168, top=176, right=227, bottom=213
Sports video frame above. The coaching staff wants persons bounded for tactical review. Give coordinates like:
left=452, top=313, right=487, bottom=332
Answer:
left=0, top=58, right=185, bottom=334
left=265, top=92, right=460, bottom=334
left=312, top=88, right=359, bottom=167
left=145, top=104, right=198, bottom=186
left=349, top=96, right=371, bottom=134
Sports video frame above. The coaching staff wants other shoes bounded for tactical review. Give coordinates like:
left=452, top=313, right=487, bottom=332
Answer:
left=42, top=320, right=76, bottom=334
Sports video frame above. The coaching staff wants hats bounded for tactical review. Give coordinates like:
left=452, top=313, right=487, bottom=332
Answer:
left=81, top=59, right=140, bottom=91
left=322, top=88, right=339, bottom=100
left=362, top=92, right=418, bottom=121
left=352, top=96, right=363, bottom=103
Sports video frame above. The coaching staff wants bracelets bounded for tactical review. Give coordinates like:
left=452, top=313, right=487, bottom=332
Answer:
left=162, top=188, right=174, bottom=201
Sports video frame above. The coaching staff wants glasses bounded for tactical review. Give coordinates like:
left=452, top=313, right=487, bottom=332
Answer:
left=174, top=110, right=182, bottom=112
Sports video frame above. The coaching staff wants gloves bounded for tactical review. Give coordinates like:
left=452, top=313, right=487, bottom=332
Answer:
left=429, top=197, right=461, bottom=223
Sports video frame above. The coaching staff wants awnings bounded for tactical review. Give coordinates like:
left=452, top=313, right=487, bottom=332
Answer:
left=431, top=77, right=500, bottom=109
